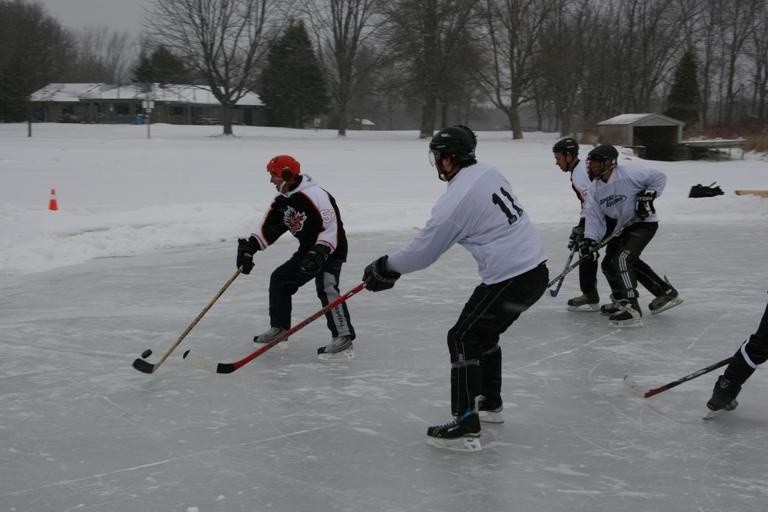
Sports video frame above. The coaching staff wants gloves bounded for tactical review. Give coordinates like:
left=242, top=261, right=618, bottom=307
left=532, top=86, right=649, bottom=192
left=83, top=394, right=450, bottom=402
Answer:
left=635, top=189, right=657, bottom=220
left=567, top=230, right=584, bottom=252
left=300, top=244, right=331, bottom=275
left=579, top=237, right=599, bottom=264
left=361, top=255, right=402, bottom=292
left=237, top=235, right=260, bottom=275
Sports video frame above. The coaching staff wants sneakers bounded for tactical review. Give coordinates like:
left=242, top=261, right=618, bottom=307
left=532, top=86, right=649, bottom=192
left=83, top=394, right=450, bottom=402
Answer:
left=323, top=336, right=353, bottom=354
left=475, top=394, right=504, bottom=414
left=427, top=411, right=482, bottom=440
left=708, top=375, right=742, bottom=411
left=567, top=290, right=600, bottom=306
left=601, top=301, right=625, bottom=313
left=609, top=304, right=642, bottom=321
left=255, top=327, right=289, bottom=344
left=649, top=286, right=678, bottom=311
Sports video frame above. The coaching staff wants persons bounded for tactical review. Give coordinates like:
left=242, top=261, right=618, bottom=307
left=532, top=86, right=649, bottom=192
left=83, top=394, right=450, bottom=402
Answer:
left=705, top=301, right=768, bottom=412
left=581, top=143, right=679, bottom=325
left=360, top=121, right=551, bottom=440
left=234, top=154, right=358, bottom=355
left=549, top=135, right=681, bottom=311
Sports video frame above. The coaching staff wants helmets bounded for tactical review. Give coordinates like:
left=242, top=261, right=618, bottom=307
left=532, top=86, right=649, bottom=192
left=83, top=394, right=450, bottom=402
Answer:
left=267, top=155, right=301, bottom=179
left=552, top=138, right=579, bottom=154
left=429, top=123, right=478, bottom=181
left=586, top=144, right=618, bottom=181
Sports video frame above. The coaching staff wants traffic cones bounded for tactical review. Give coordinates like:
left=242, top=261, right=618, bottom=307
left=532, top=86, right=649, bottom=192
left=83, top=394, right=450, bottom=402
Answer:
left=46, top=184, right=62, bottom=216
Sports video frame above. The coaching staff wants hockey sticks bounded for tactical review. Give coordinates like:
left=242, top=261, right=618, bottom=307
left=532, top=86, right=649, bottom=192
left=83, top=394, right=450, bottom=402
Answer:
left=544, top=241, right=578, bottom=299
left=623, top=357, right=734, bottom=398
left=133, top=266, right=244, bottom=374
left=182, top=276, right=366, bottom=373
